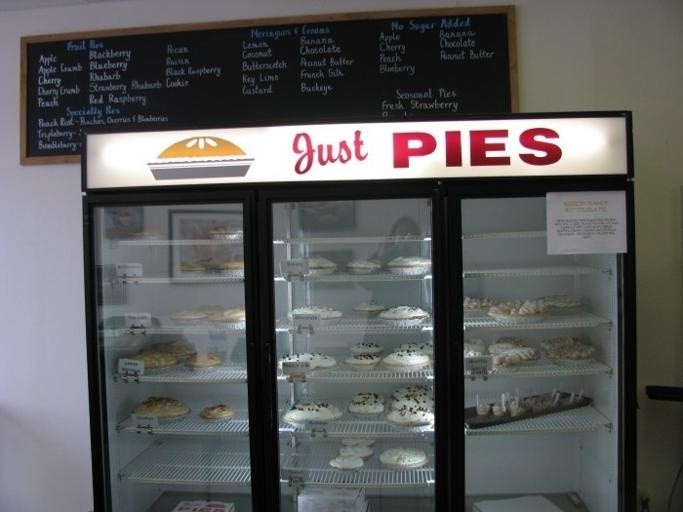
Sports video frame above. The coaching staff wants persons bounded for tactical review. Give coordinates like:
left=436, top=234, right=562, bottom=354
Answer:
left=358, top=217, right=423, bottom=394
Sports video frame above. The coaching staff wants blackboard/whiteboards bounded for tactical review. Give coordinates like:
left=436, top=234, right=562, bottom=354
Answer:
left=19, top=5, right=520, bottom=167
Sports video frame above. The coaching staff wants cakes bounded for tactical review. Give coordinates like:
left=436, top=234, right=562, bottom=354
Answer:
left=114, top=226, right=245, bottom=424
left=278, top=256, right=434, bottom=472
left=464, top=293, right=596, bottom=418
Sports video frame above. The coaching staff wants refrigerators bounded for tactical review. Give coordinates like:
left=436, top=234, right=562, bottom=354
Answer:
left=77, top=105, right=639, bottom=512
